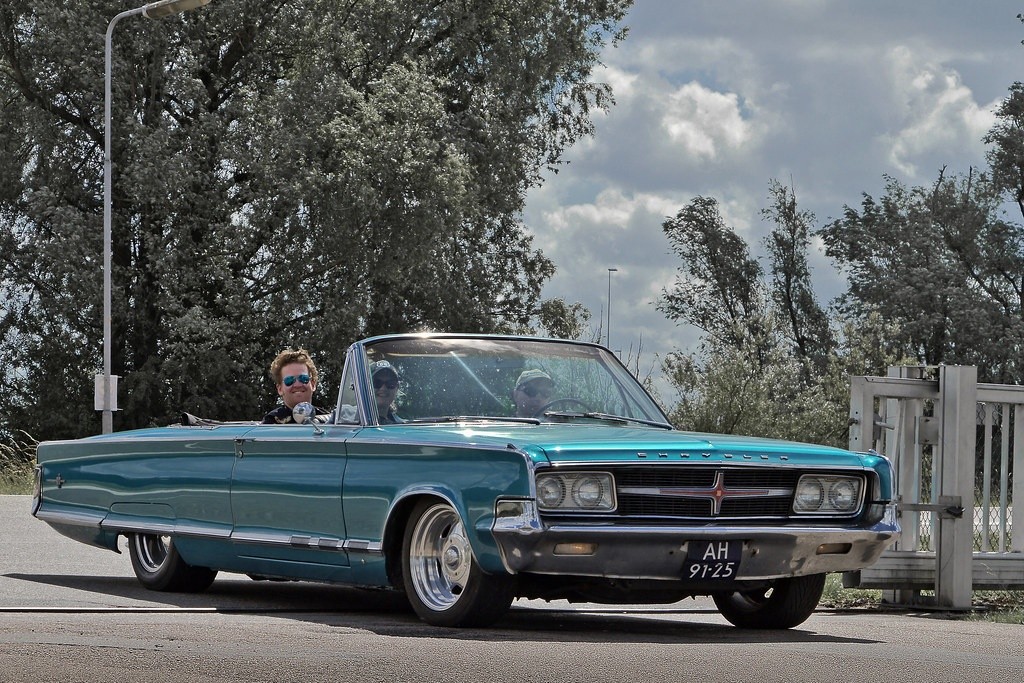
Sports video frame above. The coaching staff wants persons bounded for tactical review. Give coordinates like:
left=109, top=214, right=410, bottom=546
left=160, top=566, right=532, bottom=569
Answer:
left=508, top=368, right=555, bottom=420
left=347, top=360, right=408, bottom=424
left=260, top=350, right=330, bottom=425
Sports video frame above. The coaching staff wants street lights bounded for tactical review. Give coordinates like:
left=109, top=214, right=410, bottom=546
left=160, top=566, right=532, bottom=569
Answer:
left=606, top=267, right=618, bottom=349
left=615, top=348, right=621, bottom=360
left=97, top=0, right=214, bottom=436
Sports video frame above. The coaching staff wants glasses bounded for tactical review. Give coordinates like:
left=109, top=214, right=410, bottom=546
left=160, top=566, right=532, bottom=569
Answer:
left=517, top=386, right=551, bottom=398
left=281, top=375, right=311, bottom=386
left=372, top=378, right=398, bottom=389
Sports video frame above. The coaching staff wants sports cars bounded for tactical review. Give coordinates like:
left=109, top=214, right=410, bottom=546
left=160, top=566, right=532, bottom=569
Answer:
left=31, top=331, right=904, bottom=630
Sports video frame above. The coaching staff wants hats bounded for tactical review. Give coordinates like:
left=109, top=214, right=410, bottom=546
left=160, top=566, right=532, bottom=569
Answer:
left=515, top=369, right=552, bottom=391
left=369, top=360, right=399, bottom=384
